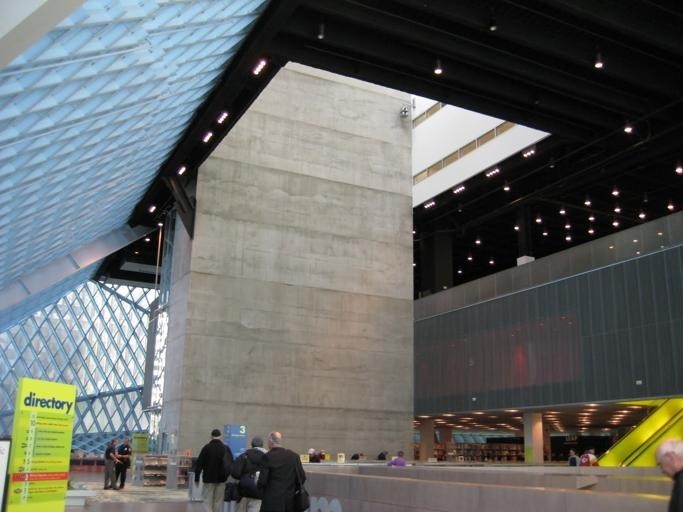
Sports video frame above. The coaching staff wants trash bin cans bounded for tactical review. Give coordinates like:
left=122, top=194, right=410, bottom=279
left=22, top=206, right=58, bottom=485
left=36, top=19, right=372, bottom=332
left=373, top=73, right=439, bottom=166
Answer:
left=189, top=472, right=203, bottom=502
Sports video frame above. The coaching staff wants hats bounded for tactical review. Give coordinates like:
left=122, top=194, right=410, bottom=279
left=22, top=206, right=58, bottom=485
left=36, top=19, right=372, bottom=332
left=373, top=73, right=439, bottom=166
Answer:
left=211, top=429, right=221, bottom=437
left=308, top=448, right=316, bottom=454
left=251, top=436, right=264, bottom=447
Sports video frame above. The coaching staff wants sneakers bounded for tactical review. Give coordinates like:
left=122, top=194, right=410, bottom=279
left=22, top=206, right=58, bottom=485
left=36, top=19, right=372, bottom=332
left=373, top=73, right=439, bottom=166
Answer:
left=103, top=484, right=124, bottom=491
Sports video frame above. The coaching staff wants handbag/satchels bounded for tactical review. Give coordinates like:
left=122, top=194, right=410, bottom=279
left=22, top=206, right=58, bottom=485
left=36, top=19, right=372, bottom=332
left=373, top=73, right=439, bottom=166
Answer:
left=224, top=482, right=243, bottom=503
left=294, top=488, right=309, bottom=510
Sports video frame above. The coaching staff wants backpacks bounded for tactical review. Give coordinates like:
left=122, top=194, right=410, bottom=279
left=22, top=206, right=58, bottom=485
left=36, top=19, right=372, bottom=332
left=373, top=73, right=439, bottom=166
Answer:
left=239, top=452, right=265, bottom=488
left=223, top=445, right=234, bottom=474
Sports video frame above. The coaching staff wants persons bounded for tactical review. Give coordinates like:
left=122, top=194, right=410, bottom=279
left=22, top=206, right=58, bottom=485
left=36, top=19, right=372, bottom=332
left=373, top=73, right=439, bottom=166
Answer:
left=107, top=435, right=131, bottom=489
left=194, top=428, right=233, bottom=512
left=253, top=430, right=306, bottom=512
left=567, top=445, right=598, bottom=466
left=307, top=446, right=407, bottom=467
left=653, top=437, right=682, bottom=512
left=229, top=435, right=268, bottom=512
left=102, top=437, right=123, bottom=490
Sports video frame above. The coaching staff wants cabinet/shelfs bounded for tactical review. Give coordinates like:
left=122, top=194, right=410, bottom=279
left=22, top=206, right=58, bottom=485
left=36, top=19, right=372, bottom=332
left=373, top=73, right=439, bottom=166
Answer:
left=134, top=455, right=199, bottom=490
